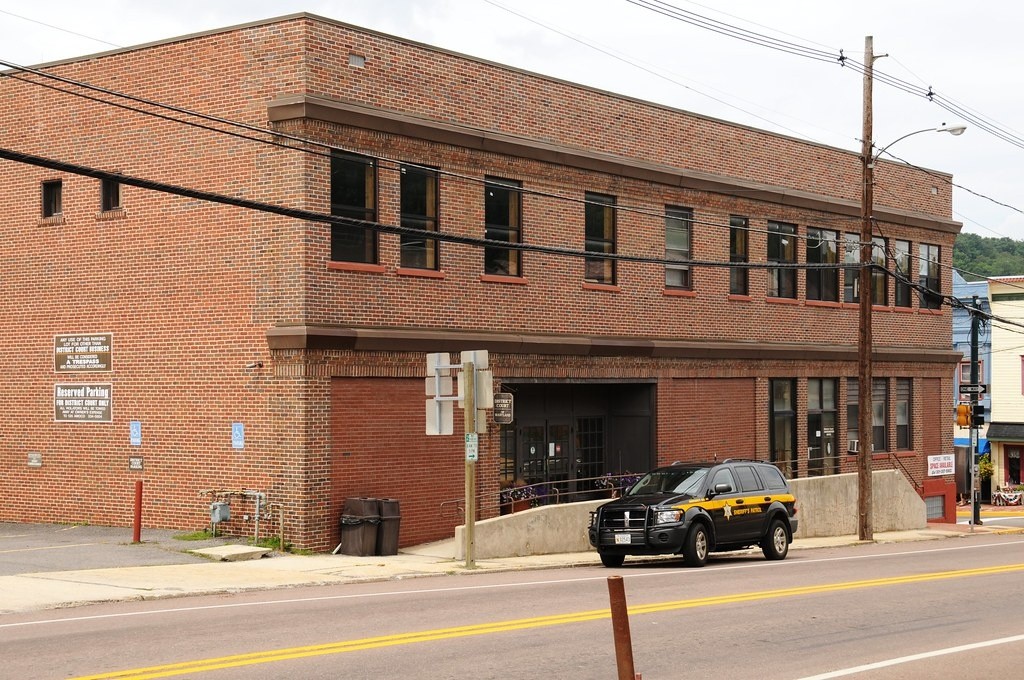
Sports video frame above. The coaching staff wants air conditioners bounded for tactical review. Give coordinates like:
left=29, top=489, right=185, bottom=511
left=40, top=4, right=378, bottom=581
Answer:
left=846, top=439, right=859, bottom=453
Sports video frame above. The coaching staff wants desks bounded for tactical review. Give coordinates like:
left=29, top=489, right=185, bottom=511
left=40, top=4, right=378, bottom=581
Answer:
left=993, top=490, right=1024, bottom=506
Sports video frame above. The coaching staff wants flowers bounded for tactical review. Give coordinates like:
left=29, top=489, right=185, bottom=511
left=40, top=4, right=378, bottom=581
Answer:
left=595, top=471, right=642, bottom=491
left=500, top=477, right=539, bottom=508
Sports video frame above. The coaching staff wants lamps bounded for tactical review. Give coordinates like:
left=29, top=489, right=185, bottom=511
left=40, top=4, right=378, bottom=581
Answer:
left=246, top=362, right=263, bottom=368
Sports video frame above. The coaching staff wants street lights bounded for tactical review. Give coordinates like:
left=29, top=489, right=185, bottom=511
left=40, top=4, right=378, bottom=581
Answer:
left=858, top=124, right=966, bottom=541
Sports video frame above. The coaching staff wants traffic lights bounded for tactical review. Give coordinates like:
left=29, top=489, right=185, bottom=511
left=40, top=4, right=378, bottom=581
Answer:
left=956, top=405, right=969, bottom=427
left=971, top=406, right=984, bottom=427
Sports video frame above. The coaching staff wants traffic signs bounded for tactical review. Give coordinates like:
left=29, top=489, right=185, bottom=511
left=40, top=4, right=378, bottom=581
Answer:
left=465, top=434, right=478, bottom=462
left=959, top=385, right=989, bottom=393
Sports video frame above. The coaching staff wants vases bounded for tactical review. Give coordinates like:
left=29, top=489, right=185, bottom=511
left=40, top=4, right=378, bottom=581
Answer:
left=504, top=498, right=532, bottom=514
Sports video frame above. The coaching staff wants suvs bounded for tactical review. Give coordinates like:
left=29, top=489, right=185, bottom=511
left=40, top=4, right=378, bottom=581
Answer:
left=587, top=454, right=800, bottom=567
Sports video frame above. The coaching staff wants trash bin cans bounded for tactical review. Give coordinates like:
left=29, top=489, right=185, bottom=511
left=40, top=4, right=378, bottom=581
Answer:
left=377, top=498, right=401, bottom=556
left=341, top=497, right=379, bottom=556
